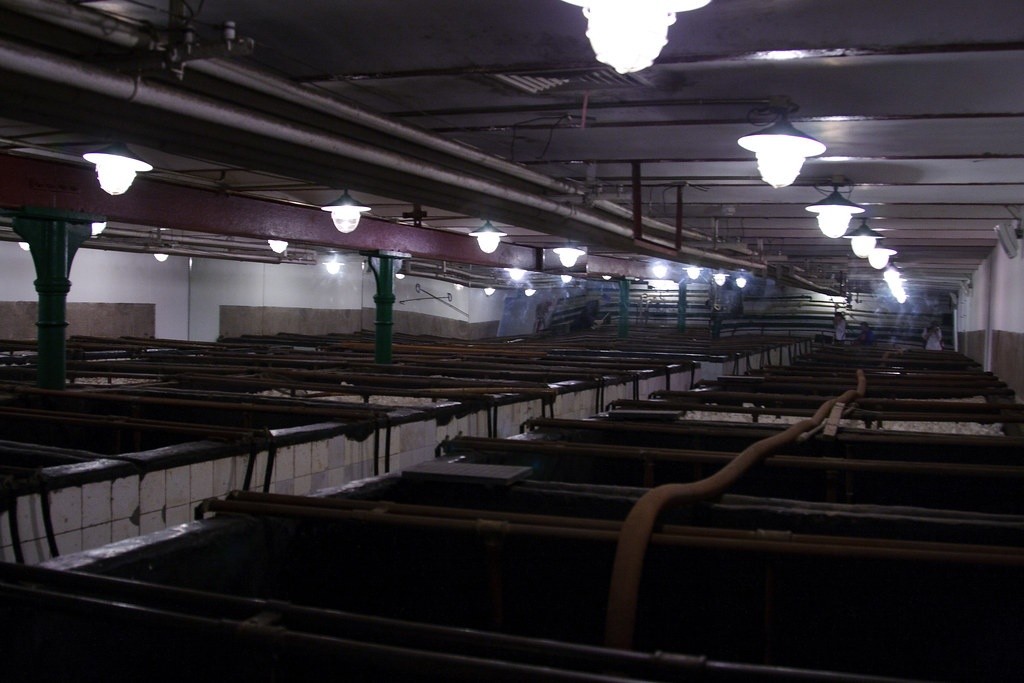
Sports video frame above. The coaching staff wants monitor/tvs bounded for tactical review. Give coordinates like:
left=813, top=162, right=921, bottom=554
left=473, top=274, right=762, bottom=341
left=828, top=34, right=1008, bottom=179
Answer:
left=950, top=292, right=958, bottom=305
left=961, top=281, right=970, bottom=295
left=994, top=223, right=1018, bottom=259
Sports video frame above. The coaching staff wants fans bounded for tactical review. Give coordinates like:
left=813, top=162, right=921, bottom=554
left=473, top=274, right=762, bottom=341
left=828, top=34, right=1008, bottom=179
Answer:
left=959, top=281, right=973, bottom=296
left=949, top=292, right=959, bottom=305
left=994, top=223, right=1024, bottom=259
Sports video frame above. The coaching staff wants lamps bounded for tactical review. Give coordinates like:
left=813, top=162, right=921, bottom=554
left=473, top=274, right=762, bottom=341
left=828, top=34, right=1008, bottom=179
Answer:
left=553, top=237, right=586, bottom=268
left=320, top=190, right=372, bottom=233
left=468, top=220, right=507, bottom=254
left=82, top=141, right=154, bottom=196
left=843, top=217, right=888, bottom=259
left=804, top=175, right=866, bottom=238
left=323, top=253, right=345, bottom=276
left=684, top=265, right=704, bottom=280
left=653, top=261, right=668, bottom=279
left=883, top=260, right=905, bottom=280
left=736, top=271, right=749, bottom=289
left=869, top=238, right=897, bottom=270
left=712, top=269, right=730, bottom=286
left=734, top=100, right=828, bottom=189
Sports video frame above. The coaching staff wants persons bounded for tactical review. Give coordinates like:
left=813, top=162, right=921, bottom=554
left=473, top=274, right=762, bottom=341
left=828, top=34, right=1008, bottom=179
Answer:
left=921, top=320, right=943, bottom=350
left=705, top=299, right=724, bottom=340
left=832, top=312, right=846, bottom=344
left=857, top=322, right=877, bottom=346
left=568, top=300, right=604, bottom=332
